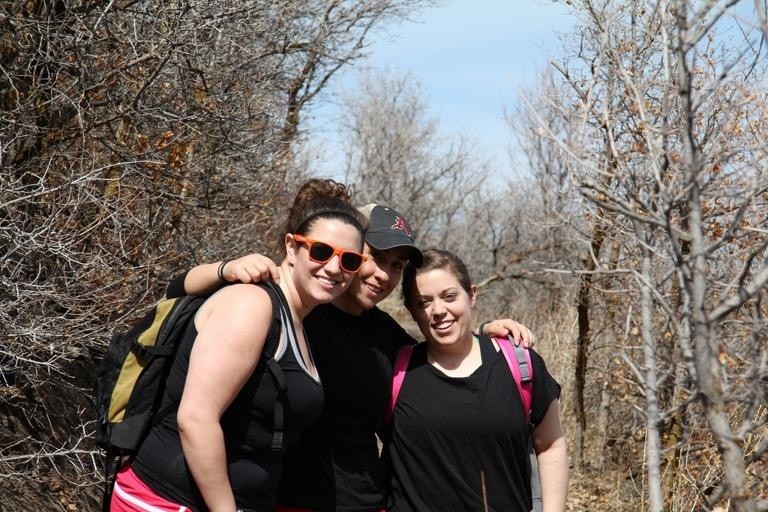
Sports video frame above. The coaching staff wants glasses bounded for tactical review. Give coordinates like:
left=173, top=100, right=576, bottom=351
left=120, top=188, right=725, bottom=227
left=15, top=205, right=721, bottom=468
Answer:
left=294, top=234, right=367, bottom=274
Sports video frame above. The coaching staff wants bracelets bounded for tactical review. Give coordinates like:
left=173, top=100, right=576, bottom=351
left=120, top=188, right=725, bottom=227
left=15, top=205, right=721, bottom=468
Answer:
left=217, top=257, right=233, bottom=285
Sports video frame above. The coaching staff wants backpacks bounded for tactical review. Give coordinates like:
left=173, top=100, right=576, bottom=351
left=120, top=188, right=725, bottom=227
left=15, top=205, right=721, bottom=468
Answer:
left=96, top=278, right=282, bottom=455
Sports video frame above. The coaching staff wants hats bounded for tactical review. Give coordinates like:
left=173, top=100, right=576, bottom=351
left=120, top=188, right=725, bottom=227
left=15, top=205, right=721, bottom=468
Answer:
left=356, top=203, right=425, bottom=270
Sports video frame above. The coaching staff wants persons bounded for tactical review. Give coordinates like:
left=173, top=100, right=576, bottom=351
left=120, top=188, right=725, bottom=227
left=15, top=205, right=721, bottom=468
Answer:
left=109, top=176, right=371, bottom=512
left=162, top=201, right=537, bottom=512
left=377, top=249, right=568, bottom=512
